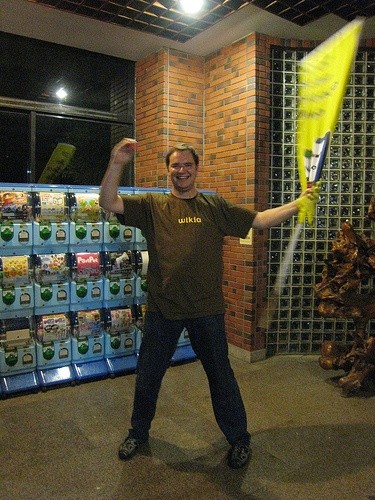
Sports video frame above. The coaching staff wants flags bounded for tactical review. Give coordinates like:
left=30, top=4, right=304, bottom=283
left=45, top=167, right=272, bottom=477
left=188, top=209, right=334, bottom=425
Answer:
left=35, top=143, right=76, bottom=184
left=293, top=16, right=365, bottom=225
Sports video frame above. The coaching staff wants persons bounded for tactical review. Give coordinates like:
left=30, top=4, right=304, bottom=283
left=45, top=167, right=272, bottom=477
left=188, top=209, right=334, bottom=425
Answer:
left=98, top=135, right=321, bottom=470
